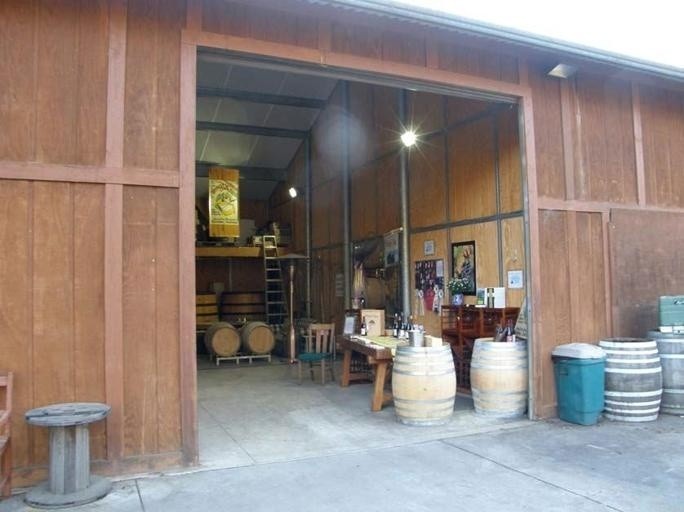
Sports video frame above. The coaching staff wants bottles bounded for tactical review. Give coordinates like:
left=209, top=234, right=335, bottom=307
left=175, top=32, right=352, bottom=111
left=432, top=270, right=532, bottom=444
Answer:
left=359, top=291, right=366, bottom=308
left=393, top=312, right=423, bottom=340
left=495, top=317, right=516, bottom=342
left=361, top=317, right=366, bottom=335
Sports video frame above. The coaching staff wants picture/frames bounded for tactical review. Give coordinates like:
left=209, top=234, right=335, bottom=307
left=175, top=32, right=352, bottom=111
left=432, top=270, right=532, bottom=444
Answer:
left=452, top=240, right=477, bottom=296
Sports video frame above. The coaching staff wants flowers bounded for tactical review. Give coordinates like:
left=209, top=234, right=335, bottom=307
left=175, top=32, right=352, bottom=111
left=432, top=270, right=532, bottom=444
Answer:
left=448, top=276, right=469, bottom=294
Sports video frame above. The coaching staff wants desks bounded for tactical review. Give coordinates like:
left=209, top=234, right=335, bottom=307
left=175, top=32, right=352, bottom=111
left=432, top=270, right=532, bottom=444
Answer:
left=336, top=334, right=394, bottom=411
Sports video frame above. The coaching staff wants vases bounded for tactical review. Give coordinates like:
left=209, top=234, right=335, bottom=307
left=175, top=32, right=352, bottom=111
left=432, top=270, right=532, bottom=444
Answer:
left=452, top=293, right=464, bottom=306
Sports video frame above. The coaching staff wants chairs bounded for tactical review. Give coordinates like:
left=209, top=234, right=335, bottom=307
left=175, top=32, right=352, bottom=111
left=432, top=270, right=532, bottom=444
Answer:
left=298, top=323, right=336, bottom=385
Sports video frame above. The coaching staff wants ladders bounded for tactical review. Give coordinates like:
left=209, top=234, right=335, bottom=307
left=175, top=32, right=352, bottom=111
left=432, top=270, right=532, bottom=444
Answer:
left=263, top=235, right=291, bottom=327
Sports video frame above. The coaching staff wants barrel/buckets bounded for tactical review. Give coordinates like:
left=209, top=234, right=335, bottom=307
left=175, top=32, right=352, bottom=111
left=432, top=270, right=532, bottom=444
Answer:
left=597, top=338, right=662, bottom=422
left=646, top=327, right=684, bottom=417
left=203, top=321, right=240, bottom=357
left=238, top=321, right=276, bottom=355
left=471, top=336, right=529, bottom=423
left=195, top=291, right=219, bottom=325
left=219, top=289, right=268, bottom=332
left=391, top=342, right=458, bottom=427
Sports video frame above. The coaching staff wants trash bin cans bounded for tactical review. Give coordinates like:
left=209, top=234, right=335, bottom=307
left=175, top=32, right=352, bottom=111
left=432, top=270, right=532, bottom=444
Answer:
left=552, top=342, right=607, bottom=426
